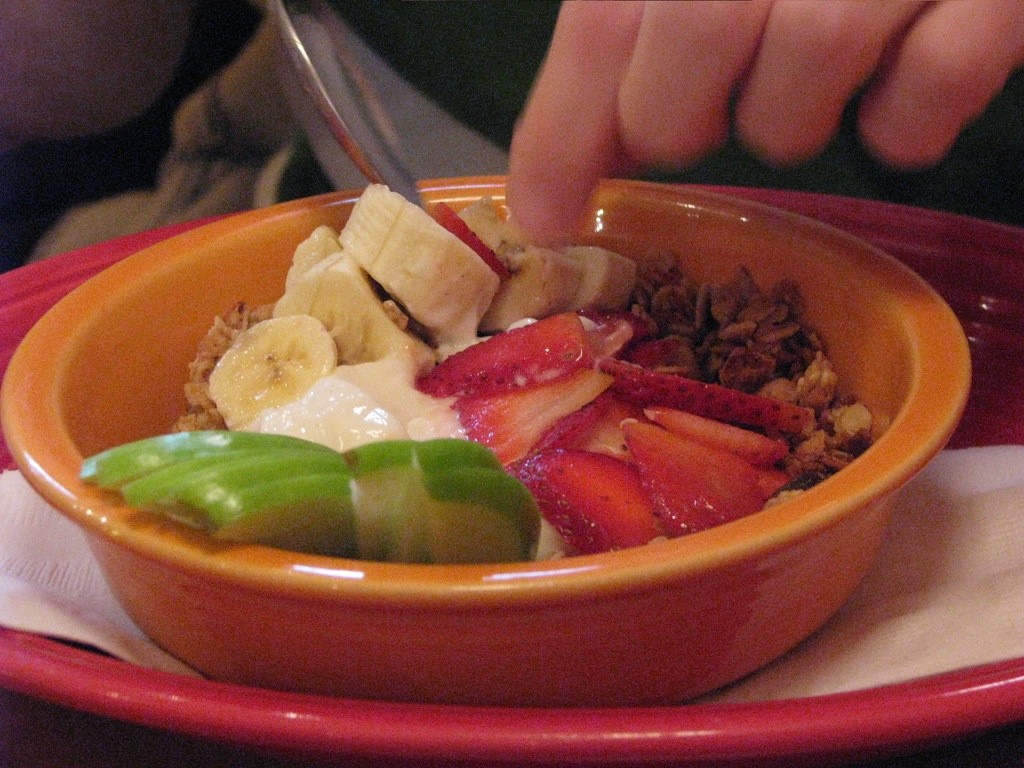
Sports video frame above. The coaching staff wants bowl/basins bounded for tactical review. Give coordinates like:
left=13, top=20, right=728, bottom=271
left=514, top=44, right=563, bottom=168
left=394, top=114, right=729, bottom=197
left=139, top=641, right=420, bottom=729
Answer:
left=0, top=174, right=974, bottom=710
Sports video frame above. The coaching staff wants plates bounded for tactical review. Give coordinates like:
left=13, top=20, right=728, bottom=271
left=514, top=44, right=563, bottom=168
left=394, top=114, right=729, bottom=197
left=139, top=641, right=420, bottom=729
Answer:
left=0, top=179, right=1024, bottom=766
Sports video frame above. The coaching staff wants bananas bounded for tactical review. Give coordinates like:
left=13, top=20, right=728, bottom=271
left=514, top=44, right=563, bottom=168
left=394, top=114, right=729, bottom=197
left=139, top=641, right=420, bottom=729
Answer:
left=209, top=181, right=636, bottom=435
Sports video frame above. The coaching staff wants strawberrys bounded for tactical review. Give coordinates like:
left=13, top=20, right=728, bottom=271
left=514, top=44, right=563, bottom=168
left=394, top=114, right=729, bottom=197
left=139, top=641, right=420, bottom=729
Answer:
left=431, top=198, right=506, bottom=277
left=414, top=308, right=820, bottom=554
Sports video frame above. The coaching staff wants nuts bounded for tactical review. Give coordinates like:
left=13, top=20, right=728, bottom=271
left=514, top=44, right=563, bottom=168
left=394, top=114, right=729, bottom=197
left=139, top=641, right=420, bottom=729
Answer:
left=172, top=299, right=407, bottom=436
left=622, top=247, right=875, bottom=493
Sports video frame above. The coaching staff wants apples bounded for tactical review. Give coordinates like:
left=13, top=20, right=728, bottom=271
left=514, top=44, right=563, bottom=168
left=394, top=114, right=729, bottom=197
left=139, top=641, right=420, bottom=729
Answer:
left=74, top=425, right=543, bottom=565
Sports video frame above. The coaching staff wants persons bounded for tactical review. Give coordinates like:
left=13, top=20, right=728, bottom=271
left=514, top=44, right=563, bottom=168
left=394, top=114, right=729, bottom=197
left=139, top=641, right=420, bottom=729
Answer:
left=0, top=1, right=1024, bottom=227
